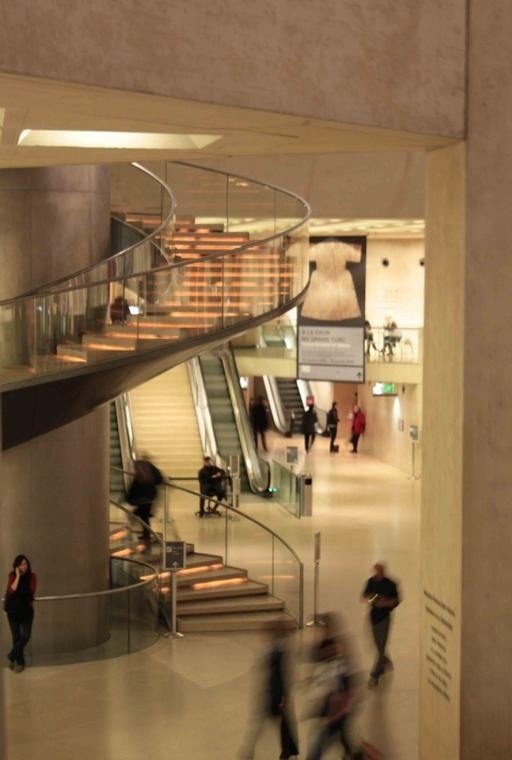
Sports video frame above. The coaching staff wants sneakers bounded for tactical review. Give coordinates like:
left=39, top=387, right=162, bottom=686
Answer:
left=7, top=656, right=24, bottom=673
left=138, top=535, right=150, bottom=540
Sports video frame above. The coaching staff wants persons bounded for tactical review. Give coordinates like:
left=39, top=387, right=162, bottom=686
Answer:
left=380, top=314, right=401, bottom=356
left=297, top=611, right=364, bottom=760
left=126, top=456, right=164, bottom=541
left=301, top=404, right=318, bottom=454
left=349, top=406, right=366, bottom=453
left=238, top=620, right=300, bottom=760
left=326, top=401, right=339, bottom=453
left=359, top=563, right=400, bottom=689
left=3, top=555, right=37, bottom=673
left=364, top=320, right=378, bottom=355
left=251, top=395, right=268, bottom=452
left=198, top=457, right=225, bottom=514
